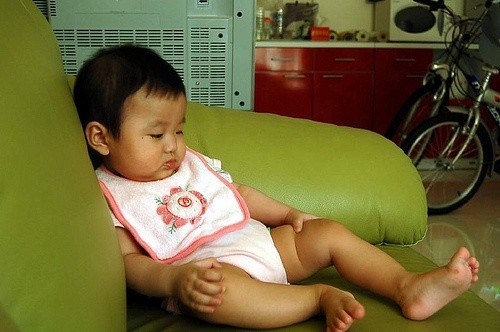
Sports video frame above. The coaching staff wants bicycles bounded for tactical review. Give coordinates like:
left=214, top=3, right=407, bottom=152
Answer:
left=365, top=0, right=500, bottom=216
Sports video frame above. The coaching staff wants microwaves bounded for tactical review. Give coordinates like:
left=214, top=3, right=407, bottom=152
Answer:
left=374, top=0, right=464, bottom=43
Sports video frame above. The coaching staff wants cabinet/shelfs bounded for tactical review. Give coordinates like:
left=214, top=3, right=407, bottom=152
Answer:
left=255, top=42, right=498, bottom=173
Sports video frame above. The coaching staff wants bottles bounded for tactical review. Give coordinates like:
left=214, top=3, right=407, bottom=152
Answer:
left=301, top=19, right=311, bottom=40
left=276, top=9, right=285, bottom=35
left=256, top=6, right=264, bottom=41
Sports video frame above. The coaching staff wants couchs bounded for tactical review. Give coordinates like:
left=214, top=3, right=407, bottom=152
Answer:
left=1, top=1, right=500, bottom=332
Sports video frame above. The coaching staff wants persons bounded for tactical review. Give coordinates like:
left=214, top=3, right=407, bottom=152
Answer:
left=72, top=44, right=481, bottom=332
